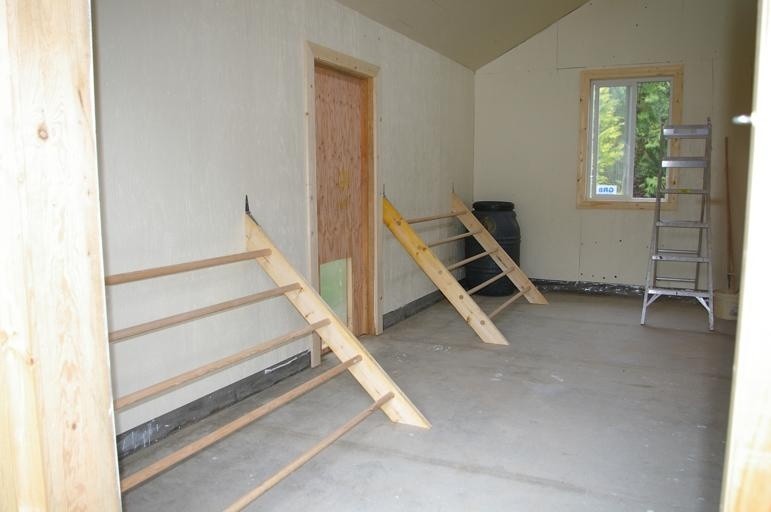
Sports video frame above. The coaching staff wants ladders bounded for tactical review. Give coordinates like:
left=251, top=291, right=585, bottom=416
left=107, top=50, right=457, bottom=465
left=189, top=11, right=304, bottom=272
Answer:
left=640, top=116, right=715, bottom=330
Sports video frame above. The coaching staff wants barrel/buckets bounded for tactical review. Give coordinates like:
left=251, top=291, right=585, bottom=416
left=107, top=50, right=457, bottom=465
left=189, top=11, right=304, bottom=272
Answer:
left=466, top=202, right=522, bottom=296
